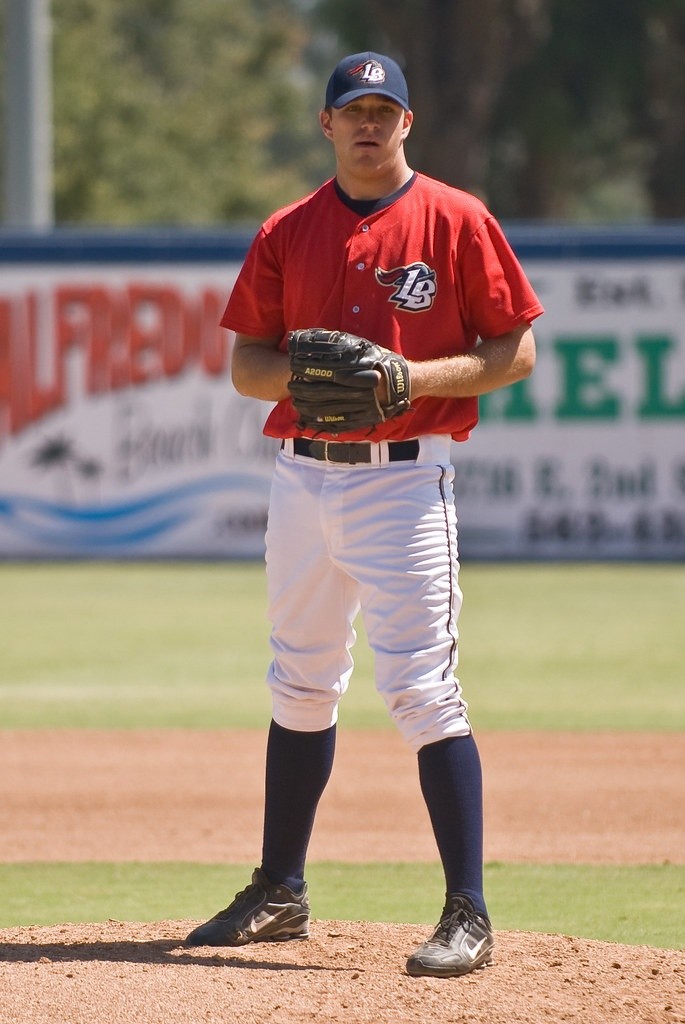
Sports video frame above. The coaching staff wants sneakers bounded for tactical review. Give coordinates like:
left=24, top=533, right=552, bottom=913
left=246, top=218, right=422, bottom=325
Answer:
left=183, top=867, right=310, bottom=946
left=405, top=892, right=494, bottom=978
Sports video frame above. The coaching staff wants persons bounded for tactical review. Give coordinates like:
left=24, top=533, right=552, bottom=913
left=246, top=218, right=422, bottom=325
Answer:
left=185, top=48, right=546, bottom=976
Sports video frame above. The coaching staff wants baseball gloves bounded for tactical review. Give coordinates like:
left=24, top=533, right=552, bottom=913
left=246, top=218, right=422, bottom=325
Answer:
left=285, top=327, right=411, bottom=435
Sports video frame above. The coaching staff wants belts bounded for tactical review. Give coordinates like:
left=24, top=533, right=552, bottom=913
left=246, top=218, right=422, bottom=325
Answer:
left=279, top=437, right=418, bottom=464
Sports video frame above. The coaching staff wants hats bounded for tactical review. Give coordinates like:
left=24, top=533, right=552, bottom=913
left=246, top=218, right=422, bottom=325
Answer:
left=324, top=51, right=408, bottom=111
